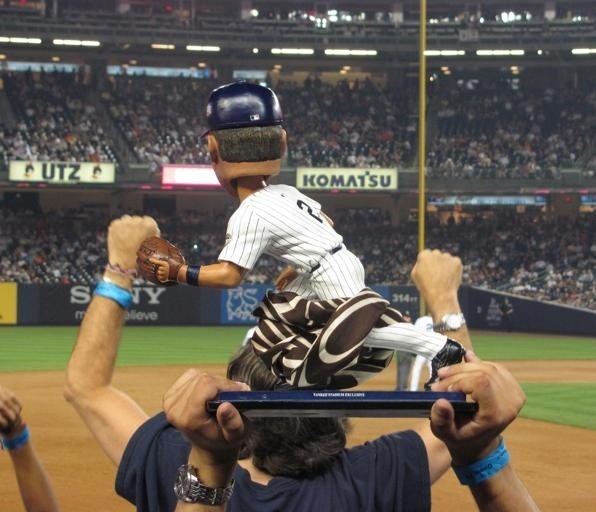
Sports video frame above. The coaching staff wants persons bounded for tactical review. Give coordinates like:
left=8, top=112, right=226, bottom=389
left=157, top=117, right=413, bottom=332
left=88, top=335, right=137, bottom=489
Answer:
left=57, top=212, right=479, bottom=512
left=1, top=0, right=595, bottom=313
left=392, top=308, right=417, bottom=391
left=136, top=77, right=465, bottom=395
left=0, top=387, right=58, bottom=511
left=159, top=347, right=544, bottom=511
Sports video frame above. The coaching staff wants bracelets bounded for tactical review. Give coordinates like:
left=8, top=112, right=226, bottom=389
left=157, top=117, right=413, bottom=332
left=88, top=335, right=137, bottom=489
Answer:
left=449, top=437, right=512, bottom=487
left=2, top=425, right=29, bottom=451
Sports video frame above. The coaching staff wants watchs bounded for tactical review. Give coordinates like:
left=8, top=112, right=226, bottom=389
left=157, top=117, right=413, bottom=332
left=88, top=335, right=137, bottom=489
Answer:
left=432, top=312, right=467, bottom=336
left=171, top=463, right=236, bottom=506
